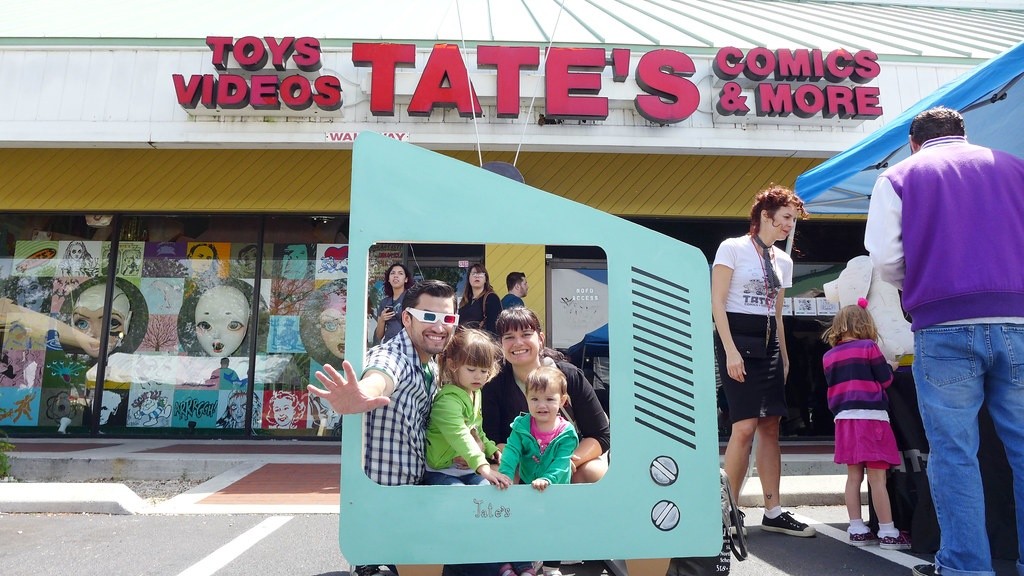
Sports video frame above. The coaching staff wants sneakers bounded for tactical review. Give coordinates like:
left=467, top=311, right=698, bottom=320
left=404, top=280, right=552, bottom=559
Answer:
left=723, top=509, right=747, bottom=536
left=761, top=511, right=816, bottom=538
left=850, top=528, right=878, bottom=546
left=879, top=529, right=912, bottom=548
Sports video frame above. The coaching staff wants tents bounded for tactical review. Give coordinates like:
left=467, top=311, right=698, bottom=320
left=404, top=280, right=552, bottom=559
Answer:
left=785, top=39, right=1024, bottom=253
left=568, top=324, right=610, bottom=371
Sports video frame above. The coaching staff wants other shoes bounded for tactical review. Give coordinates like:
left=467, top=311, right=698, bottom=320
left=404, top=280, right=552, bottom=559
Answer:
left=351, top=564, right=385, bottom=576
left=912, top=564, right=942, bottom=576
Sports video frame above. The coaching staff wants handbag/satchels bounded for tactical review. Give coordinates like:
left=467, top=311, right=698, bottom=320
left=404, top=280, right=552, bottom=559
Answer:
left=676, top=468, right=748, bottom=576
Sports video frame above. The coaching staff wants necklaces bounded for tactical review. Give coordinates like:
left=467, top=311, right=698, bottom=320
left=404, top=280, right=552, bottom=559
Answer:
left=752, top=238, right=776, bottom=347
left=391, top=289, right=405, bottom=304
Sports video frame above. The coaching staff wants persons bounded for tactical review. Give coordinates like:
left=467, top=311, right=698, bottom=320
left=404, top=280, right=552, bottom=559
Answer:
left=423, top=328, right=514, bottom=576
left=865, top=107, right=1024, bottom=576
left=497, top=365, right=580, bottom=576
left=372, top=264, right=417, bottom=346
left=307, top=279, right=460, bottom=534
left=211, top=358, right=239, bottom=382
left=501, top=272, right=529, bottom=311
left=458, top=263, right=504, bottom=339
left=481, top=303, right=609, bottom=576
left=821, top=296, right=912, bottom=551
left=710, top=185, right=817, bottom=537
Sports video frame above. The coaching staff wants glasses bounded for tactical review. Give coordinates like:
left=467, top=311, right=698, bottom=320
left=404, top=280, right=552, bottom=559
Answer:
left=406, top=308, right=460, bottom=325
left=470, top=272, right=486, bottom=276
